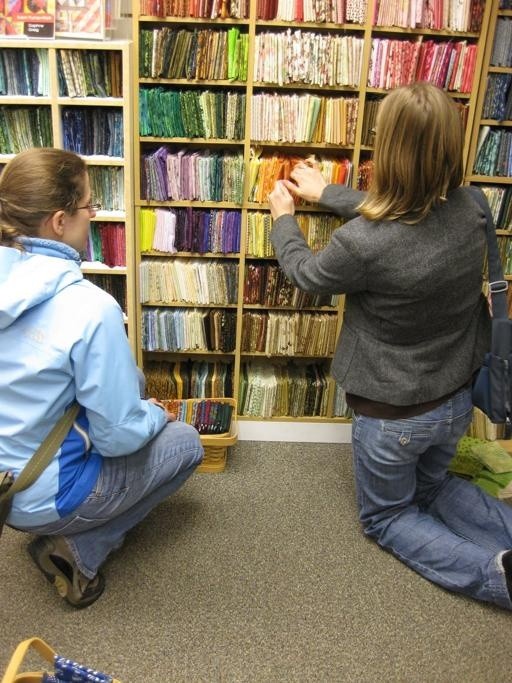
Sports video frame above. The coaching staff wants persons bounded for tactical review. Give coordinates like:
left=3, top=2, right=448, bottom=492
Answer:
left=265, top=84, right=512, bottom=610
left=0, top=148, right=205, bottom=610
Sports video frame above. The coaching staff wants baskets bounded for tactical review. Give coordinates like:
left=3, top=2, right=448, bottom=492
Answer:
left=158, top=398, right=238, bottom=471
left=2, top=638, right=120, bottom=682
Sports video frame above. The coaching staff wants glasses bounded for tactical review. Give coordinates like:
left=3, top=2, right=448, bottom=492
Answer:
left=63, top=204, right=102, bottom=213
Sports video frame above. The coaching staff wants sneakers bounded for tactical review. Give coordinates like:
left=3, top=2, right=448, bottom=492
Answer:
left=27, top=534, right=103, bottom=608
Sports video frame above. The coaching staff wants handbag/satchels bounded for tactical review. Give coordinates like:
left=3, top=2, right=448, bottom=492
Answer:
left=1, top=470, right=14, bottom=538
left=470, top=351, right=511, bottom=423
